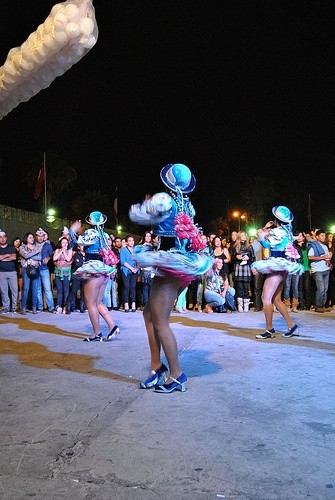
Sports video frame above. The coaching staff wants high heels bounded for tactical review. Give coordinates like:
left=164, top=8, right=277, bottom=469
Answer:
left=107, top=326, right=120, bottom=339
left=155, top=373, right=186, bottom=393
left=256, top=328, right=275, bottom=339
left=83, top=331, right=102, bottom=342
left=281, top=324, right=299, bottom=337
left=140, top=363, right=169, bottom=389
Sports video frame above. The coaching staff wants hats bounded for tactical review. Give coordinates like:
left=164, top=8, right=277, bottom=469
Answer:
left=85, top=211, right=107, bottom=226
left=272, top=206, right=295, bottom=223
left=159, top=163, right=196, bottom=194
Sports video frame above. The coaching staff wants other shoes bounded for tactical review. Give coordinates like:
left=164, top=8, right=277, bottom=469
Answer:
left=2, top=298, right=334, bottom=315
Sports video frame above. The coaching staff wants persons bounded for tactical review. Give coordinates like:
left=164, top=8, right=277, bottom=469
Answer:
left=250, top=205, right=303, bottom=340
left=1, top=223, right=334, bottom=317
left=127, top=164, right=214, bottom=393
left=69, top=212, right=121, bottom=344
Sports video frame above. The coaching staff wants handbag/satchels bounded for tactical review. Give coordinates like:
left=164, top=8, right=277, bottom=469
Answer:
left=26, top=264, right=39, bottom=279
